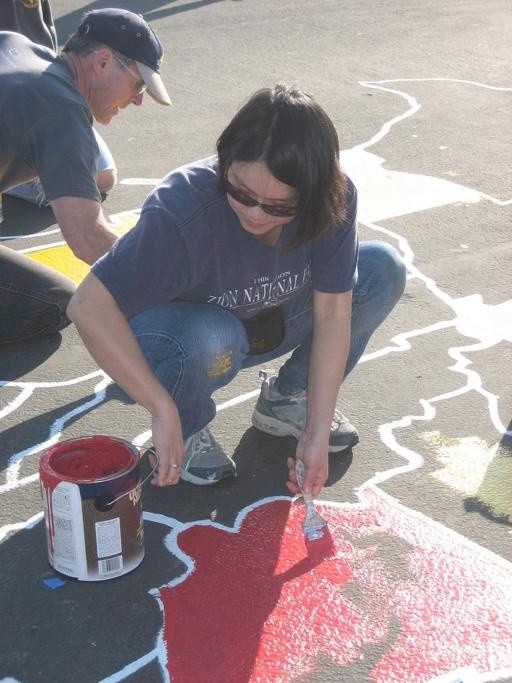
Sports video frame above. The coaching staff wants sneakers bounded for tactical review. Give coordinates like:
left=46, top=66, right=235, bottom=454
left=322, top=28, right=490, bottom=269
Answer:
left=180, top=424, right=240, bottom=489
left=251, top=372, right=361, bottom=454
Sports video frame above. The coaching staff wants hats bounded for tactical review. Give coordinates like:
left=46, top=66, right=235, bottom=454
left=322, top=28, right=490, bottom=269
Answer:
left=76, top=3, right=177, bottom=109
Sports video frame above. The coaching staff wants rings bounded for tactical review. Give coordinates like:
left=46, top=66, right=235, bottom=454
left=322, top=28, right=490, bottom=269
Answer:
left=169, top=462, right=179, bottom=469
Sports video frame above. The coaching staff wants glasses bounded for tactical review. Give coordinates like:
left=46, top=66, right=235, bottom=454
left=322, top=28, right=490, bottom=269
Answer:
left=220, top=166, right=309, bottom=219
left=111, top=49, right=150, bottom=96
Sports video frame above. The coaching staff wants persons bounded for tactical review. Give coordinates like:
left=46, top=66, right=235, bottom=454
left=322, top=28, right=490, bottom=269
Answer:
left=65, top=84, right=407, bottom=508
left=0, top=0, right=119, bottom=220
left=0, top=5, right=174, bottom=381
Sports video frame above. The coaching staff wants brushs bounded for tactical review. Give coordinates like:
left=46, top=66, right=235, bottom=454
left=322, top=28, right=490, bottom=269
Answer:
left=294, top=458, right=335, bottom=565
